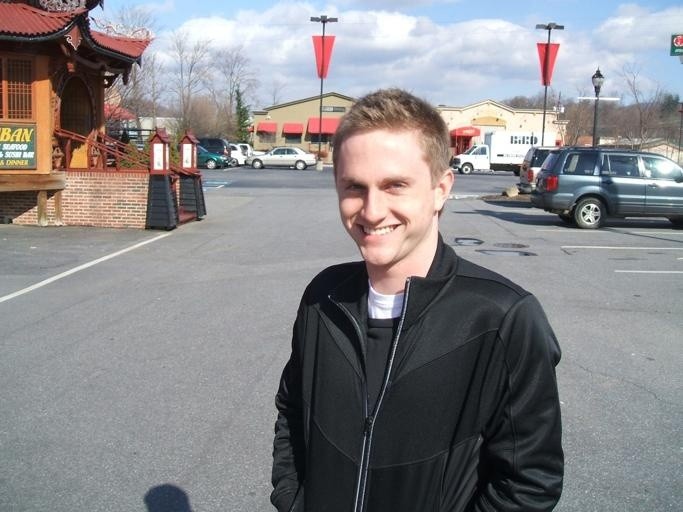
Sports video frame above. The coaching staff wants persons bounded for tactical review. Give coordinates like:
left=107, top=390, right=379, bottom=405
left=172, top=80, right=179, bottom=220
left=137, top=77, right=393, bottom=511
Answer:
left=268, top=86, right=567, bottom=511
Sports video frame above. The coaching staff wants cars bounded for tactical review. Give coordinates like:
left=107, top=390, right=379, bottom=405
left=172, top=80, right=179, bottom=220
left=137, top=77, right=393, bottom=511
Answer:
left=197, top=138, right=265, bottom=169
left=516, top=147, right=558, bottom=194
left=530, top=148, right=682, bottom=229
left=244, top=146, right=316, bottom=171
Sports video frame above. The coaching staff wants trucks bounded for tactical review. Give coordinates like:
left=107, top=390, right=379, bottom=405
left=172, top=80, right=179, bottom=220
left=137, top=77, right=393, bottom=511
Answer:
left=451, top=130, right=557, bottom=176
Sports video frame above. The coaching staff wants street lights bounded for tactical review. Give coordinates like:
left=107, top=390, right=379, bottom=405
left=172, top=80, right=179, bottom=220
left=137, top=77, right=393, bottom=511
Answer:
left=591, top=68, right=603, bottom=150
